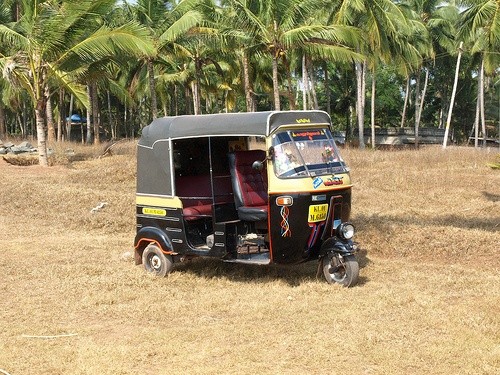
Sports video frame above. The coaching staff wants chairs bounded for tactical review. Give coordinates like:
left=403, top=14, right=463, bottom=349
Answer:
left=229, top=150, right=269, bottom=221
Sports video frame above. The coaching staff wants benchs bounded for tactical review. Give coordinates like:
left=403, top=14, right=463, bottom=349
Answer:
left=175, top=175, right=234, bottom=220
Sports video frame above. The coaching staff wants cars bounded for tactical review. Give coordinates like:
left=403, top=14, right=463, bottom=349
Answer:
left=330, top=130, right=347, bottom=145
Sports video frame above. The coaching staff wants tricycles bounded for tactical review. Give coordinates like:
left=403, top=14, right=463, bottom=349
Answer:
left=132, top=109, right=360, bottom=288
